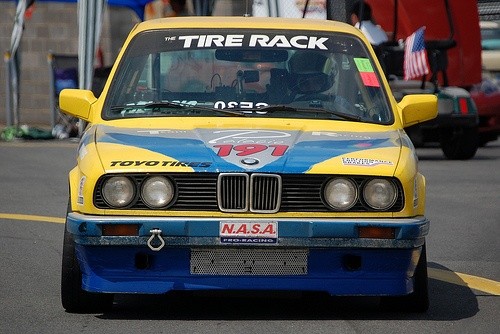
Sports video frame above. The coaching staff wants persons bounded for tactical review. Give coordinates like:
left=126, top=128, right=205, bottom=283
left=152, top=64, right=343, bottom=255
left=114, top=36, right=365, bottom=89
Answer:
left=349, top=2, right=389, bottom=45
left=278, top=50, right=368, bottom=121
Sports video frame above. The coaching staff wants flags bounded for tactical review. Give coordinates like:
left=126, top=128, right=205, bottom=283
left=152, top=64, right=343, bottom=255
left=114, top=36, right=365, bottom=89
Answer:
left=401, top=28, right=431, bottom=82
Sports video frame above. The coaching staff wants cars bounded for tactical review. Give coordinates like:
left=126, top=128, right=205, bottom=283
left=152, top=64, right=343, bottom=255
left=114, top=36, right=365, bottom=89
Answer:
left=58, top=16, right=440, bottom=314
